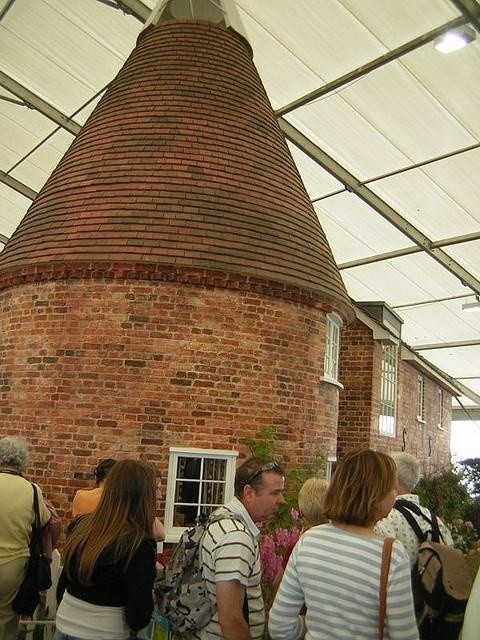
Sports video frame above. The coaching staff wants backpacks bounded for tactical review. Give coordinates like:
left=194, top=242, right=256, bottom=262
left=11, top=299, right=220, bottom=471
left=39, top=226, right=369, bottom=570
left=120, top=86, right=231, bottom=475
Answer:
left=156, top=513, right=249, bottom=637
left=392, top=498, right=473, bottom=640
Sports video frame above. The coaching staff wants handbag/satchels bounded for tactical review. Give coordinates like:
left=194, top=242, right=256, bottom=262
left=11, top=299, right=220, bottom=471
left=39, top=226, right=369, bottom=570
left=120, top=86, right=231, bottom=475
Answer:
left=11, top=480, right=53, bottom=617
left=56, top=563, right=68, bottom=604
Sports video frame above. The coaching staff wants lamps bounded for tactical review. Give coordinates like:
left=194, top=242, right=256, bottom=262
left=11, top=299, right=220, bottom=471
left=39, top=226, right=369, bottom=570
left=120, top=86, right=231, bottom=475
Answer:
left=434, top=22, right=475, bottom=54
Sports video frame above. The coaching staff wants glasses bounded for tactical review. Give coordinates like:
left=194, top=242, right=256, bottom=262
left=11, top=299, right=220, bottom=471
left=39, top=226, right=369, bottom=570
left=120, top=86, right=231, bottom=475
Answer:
left=245, top=461, right=278, bottom=484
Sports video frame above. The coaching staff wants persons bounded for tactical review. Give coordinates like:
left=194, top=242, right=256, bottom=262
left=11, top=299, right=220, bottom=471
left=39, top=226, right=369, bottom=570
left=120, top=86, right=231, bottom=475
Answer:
left=53, top=459, right=165, bottom=640
left=269, top=448, right=420, bottom=640
left=179, top=456, right=285, bottom=640
left=373, top=451, right=454, bottom=620
left=0, top=435, right=51, bottom=640
left=273, top=479, right=330, bottom=614
left=460, top=567, right=480, bottom=640
